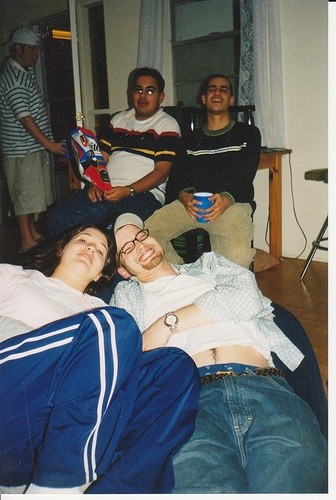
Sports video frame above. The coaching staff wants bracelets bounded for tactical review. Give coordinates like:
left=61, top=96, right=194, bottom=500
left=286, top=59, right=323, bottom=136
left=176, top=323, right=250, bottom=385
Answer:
left=126, top=186, right=137, bottom=200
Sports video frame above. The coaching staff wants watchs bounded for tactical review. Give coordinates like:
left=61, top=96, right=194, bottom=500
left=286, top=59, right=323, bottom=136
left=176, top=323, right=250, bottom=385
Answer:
left=164, top=312, right=178, bottom=332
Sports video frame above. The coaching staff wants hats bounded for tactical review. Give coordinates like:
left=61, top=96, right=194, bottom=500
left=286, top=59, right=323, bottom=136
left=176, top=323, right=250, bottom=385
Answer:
left=7, top=26, right=41, bottom=48
left=107, top=212, right=145, bottom=234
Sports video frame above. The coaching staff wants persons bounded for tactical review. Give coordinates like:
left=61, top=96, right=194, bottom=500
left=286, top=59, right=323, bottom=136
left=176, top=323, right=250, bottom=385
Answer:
left=40, top=67, right=181, bottom=302
left=143, top=74, right=261, bottom=270
left=108, top=212, right=328, bottom=495
left=0, top=223, right=202, bottom=494
left=0, top=27, right=68, bottom=253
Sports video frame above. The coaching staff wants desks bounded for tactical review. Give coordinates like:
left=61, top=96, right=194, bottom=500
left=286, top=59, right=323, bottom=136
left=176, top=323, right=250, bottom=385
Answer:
left=257, top=152, right=283, bottom=262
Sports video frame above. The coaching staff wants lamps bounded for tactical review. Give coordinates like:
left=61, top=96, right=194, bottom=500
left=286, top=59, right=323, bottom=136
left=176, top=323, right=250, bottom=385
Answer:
left=42, top=25, right=71, bottom=40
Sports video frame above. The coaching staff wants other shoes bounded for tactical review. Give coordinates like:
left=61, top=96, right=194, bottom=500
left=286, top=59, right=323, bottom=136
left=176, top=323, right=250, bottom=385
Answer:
left=36, top=231, right=58, bottom=245
left=25, top=243, right=57, bottom=264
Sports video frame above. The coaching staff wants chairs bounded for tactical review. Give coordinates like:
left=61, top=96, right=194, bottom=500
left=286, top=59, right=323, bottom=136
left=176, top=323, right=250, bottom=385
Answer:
left=15, top=105, right=328, bottom=438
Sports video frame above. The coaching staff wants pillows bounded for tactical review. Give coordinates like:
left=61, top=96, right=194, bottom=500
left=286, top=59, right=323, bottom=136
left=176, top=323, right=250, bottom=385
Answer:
left=253, top=247, right=280, bottom=273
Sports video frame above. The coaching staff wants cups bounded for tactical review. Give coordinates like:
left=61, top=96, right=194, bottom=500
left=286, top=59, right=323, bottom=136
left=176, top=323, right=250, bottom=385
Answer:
left=193, top=192, right=214, bottom=223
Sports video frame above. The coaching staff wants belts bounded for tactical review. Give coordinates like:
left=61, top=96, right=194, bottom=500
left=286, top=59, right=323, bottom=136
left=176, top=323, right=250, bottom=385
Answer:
left=200, top=368, right=285, bottom=383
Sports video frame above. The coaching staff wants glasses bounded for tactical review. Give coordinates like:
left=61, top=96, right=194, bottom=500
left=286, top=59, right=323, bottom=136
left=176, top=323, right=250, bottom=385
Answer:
left=118, top=228, right=149, bottom=268
left=132, top=87, right=161, bottom=96
left=23, top=45, right=41, bottom=52
